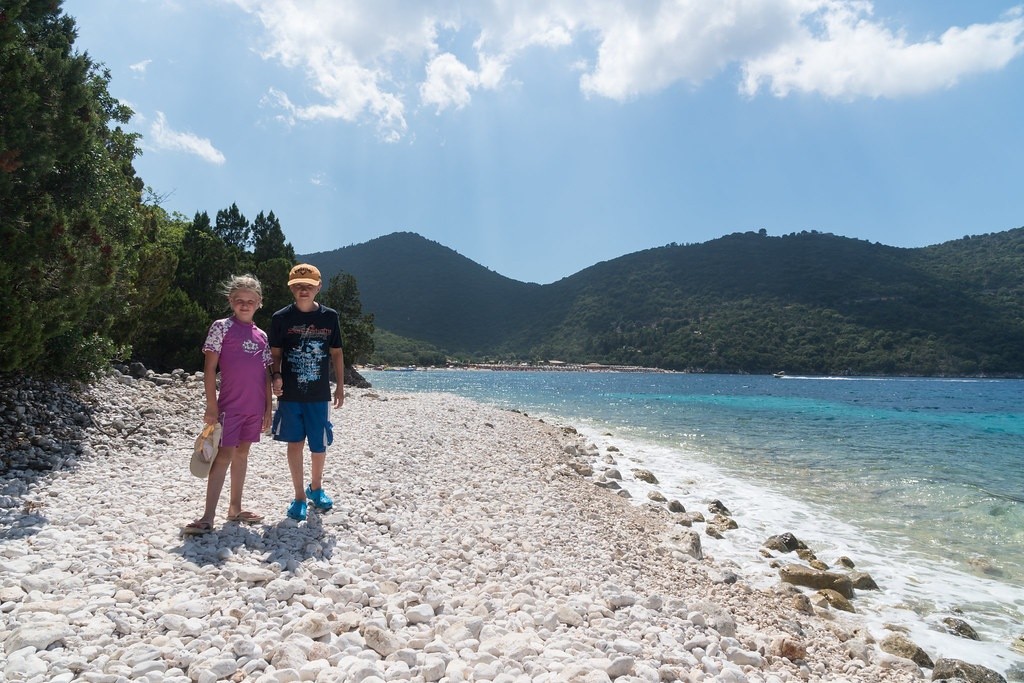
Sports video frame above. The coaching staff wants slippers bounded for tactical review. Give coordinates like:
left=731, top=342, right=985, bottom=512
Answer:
left=228, top=511, right=265, bottom=521
left=186, top=521, right=217, bottom=533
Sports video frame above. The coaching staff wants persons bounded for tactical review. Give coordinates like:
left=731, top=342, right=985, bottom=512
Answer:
left=184, top=274, right=274, bottom=534
left=270, top=263, right=344, bottom=520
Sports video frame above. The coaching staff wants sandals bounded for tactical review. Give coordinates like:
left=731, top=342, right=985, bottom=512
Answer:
left=306, top=484, right=333, bottom=509
left=287, top=499, right=308, bottom=520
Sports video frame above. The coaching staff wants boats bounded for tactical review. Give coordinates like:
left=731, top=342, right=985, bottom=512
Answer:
left=773, top=371, right=785, bottom=378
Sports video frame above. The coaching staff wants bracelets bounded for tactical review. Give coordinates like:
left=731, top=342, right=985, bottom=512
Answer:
left=272, top=371, right=280, bottom=379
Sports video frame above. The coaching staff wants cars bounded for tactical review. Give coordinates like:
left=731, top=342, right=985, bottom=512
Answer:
left=366, top=364, right=376, bottom=368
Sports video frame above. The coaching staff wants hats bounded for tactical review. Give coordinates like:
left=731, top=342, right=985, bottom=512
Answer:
left=189, top=421, right=222, bottom=479
left=287, top=263, right=322, bottom=286
